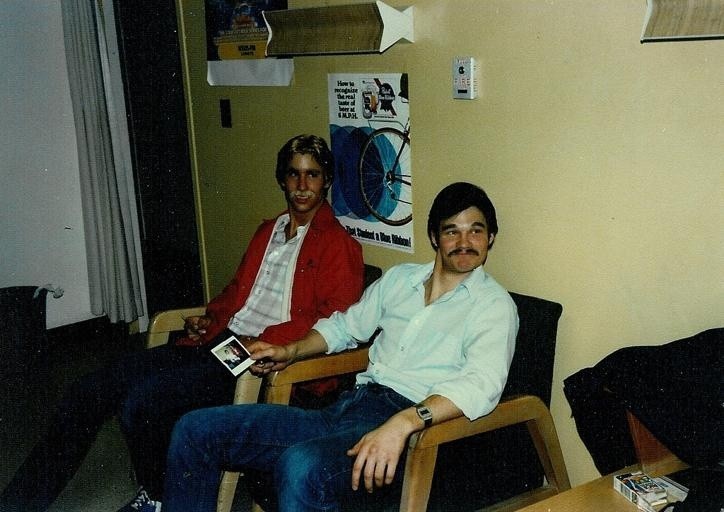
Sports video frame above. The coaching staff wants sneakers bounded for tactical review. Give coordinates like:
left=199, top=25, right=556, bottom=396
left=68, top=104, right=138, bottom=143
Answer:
left=115, top=485, right=161, bottom=512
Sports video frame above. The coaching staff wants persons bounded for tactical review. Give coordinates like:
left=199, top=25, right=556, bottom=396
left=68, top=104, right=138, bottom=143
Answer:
left=162, top=182, right=520, bottom=512
left=117, top=134, right=363, bottom=512
left=223, top=348, right=241, bottom=364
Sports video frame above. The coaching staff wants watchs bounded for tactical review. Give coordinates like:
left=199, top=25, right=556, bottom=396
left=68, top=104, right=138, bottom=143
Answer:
left=413, top=404, right=432, bottom=428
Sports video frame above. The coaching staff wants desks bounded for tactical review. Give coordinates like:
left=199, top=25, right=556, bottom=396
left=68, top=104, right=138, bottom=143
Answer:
left=515, top=454, right=692, bottom=511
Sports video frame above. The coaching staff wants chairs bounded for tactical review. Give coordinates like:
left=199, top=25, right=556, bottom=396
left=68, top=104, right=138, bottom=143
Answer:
left=261, top=290, right=571, bottom=510
left=146, top=263, right=384, bottom=509
